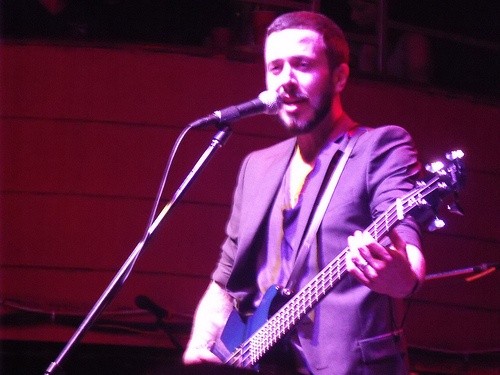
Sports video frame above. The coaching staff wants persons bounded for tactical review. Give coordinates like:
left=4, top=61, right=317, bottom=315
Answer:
left=183, top=11, right=426, bottom=375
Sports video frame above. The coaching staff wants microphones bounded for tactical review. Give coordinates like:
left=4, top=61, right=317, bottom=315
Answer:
left=189, top=91, right=282, bottom=130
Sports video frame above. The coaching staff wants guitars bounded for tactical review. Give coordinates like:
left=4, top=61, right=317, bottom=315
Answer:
left=163, top=148, right=485, bottom=375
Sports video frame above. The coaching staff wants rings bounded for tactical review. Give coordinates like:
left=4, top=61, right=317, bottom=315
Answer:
left=360, top=262, right=369, bottom=272
